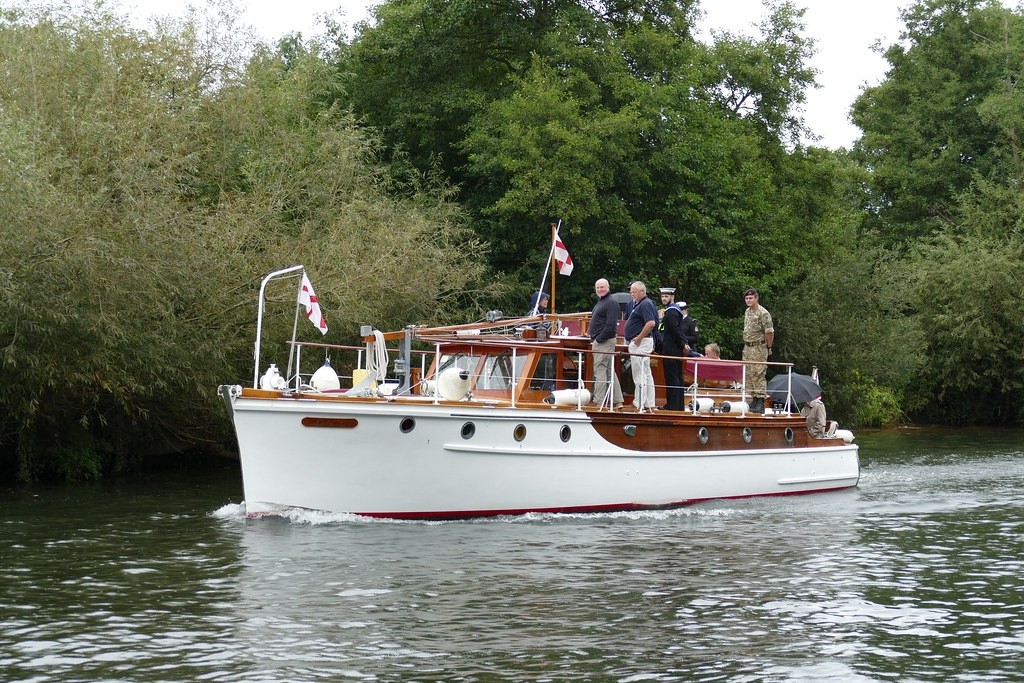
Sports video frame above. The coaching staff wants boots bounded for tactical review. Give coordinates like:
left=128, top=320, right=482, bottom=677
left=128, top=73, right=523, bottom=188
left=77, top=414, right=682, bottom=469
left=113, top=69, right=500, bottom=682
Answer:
left=748, top=397, right=765, bottom=416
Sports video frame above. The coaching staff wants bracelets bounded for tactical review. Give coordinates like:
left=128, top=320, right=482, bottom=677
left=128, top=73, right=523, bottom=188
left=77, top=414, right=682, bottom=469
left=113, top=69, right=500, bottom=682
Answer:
left=767, top=347, right=772, bottom=349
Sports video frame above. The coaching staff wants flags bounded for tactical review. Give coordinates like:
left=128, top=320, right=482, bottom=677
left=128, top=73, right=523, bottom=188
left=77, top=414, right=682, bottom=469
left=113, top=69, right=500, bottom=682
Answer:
left=298, top=270, right=329, bottom=335
left=555, top=229, right=574, bottom=276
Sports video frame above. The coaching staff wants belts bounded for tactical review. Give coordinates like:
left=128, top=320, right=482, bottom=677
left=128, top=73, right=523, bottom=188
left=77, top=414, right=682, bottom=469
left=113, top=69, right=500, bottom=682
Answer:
left=744, top=340, right=765, bottom=347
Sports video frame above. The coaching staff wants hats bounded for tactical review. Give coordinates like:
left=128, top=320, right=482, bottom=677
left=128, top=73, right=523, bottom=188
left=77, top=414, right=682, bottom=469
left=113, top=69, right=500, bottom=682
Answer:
left=676, top=301, right=687, bottom=307
left=743, top=289, right=756, bottom=297
left=659, top=287, right=677, bottom=295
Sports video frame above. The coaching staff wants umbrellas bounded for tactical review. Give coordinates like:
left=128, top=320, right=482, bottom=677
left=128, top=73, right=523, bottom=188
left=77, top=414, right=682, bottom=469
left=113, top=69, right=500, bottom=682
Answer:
left=766, top=371, right=822, bottom=405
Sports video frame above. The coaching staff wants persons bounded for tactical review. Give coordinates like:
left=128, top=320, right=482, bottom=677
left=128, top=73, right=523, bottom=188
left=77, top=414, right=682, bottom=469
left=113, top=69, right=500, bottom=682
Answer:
left=675, top=301, right=699, bottom=357
left=525, top=292, right=553, bottom=391
left=588, top=279, right=625, bottom=410
left=657, top=288, right=692, bottom=410
left=800, top=398, right=826, bottom=438
left=624, top=281, right=659, bottom=414
left=655, top=309, right=666, bottom=354
left=740, top=288, right=775, bottom=415
left=704, top=343, right=721, bottom=361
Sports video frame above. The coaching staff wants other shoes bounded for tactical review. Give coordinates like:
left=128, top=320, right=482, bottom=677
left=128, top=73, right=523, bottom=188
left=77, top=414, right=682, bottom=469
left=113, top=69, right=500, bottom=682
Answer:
left=663, top=404, right=684, bottom=411
left=590, top=402, right=602, bottom=407
left=615, top=403, right=624, bottom=408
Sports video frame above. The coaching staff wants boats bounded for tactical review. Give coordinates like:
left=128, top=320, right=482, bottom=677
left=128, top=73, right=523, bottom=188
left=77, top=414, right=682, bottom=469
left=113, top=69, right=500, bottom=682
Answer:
left=218, top=217, right=858, bottom=516
